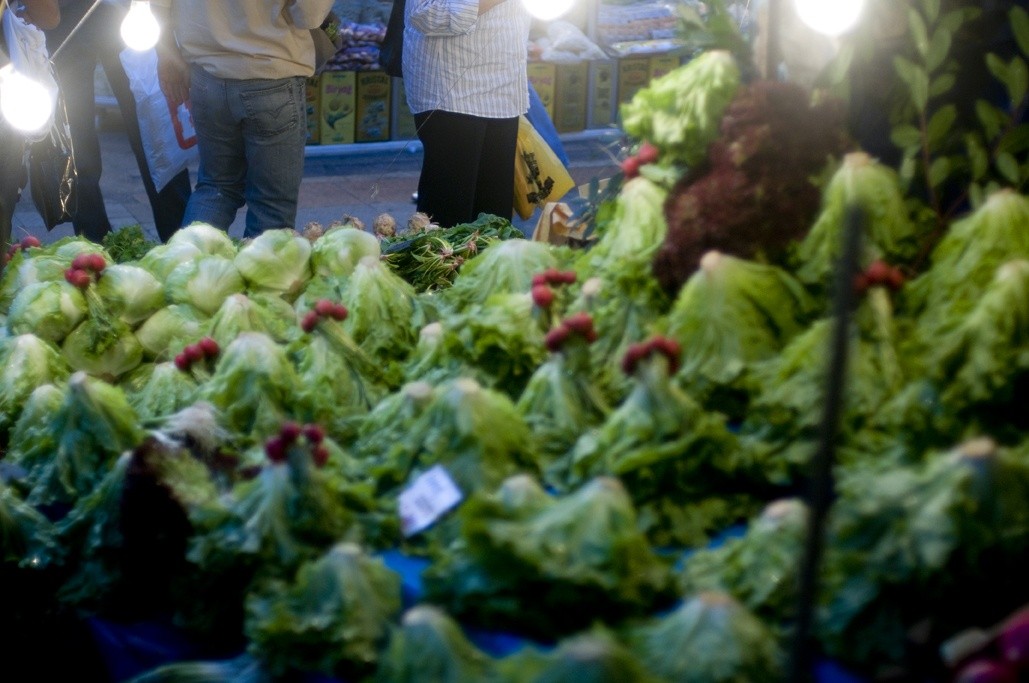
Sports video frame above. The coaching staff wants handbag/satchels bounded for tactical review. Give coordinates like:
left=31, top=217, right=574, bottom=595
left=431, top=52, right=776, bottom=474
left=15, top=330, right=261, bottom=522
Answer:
left=377, top=0, right=406, bottom=70
left=512, top=82, right=576, bottom=218
left=2, top=0, right=79, bottom=232
left=119, top=44, right=200, bottom=193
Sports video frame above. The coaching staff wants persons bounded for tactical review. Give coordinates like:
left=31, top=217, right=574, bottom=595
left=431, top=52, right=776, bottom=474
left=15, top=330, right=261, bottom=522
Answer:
left=0, top=0, right=60, bottom=257
left=151, top=0, right=340, bottom=245
left=43, top=0, right=190, bottom=245
left=402, top=0, right=529, bottom=229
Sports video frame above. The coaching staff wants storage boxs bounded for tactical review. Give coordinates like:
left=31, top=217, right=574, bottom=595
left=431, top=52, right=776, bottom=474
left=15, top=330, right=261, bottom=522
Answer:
left=306, top=56, right=678, bottom=146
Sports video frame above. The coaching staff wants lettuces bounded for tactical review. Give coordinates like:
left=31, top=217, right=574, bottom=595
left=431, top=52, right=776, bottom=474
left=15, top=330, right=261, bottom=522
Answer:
left=0, top=46, right=1029, bottom=683
